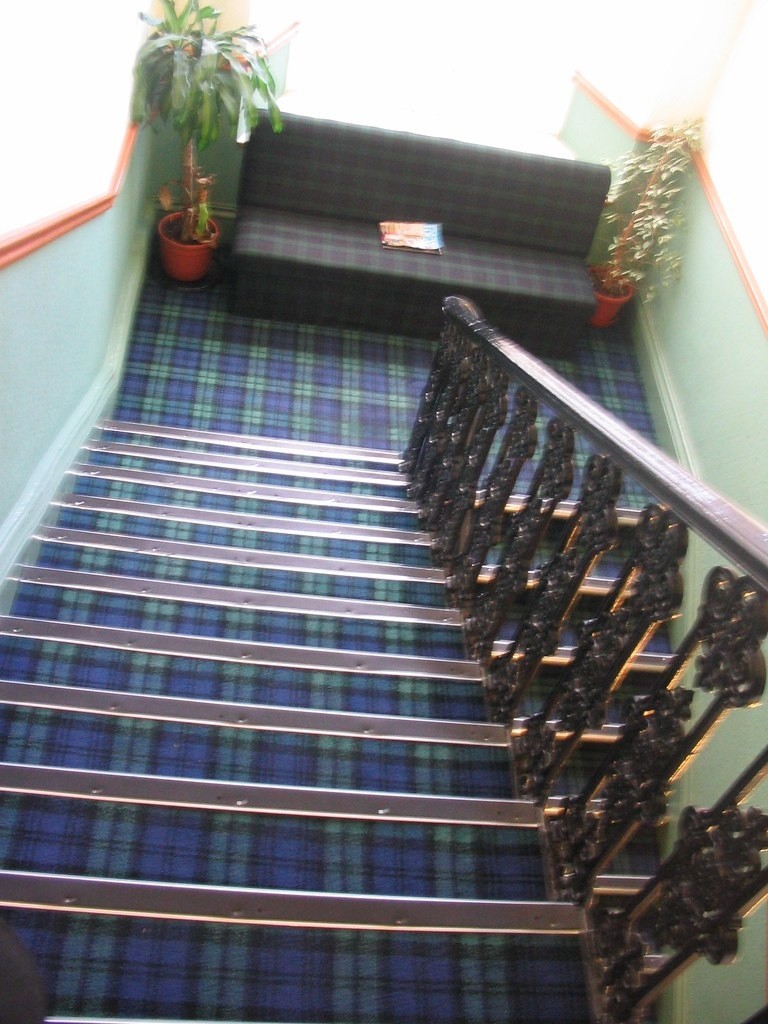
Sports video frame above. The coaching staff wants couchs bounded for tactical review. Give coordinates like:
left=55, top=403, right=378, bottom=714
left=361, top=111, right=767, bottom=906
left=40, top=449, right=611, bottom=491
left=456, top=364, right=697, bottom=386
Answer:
left=227, top=105, right=613, bottom=367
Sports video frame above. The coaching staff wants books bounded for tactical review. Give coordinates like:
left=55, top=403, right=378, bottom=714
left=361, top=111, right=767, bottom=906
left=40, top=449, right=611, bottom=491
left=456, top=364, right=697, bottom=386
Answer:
left=378, top=220, right=444, bottom=254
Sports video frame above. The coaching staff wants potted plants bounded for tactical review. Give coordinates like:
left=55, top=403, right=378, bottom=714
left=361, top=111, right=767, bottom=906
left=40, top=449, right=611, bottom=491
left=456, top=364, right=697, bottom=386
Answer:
left=130, top=2, right=282, bottom=280
left=587, top=119, right=703, bottom=327
